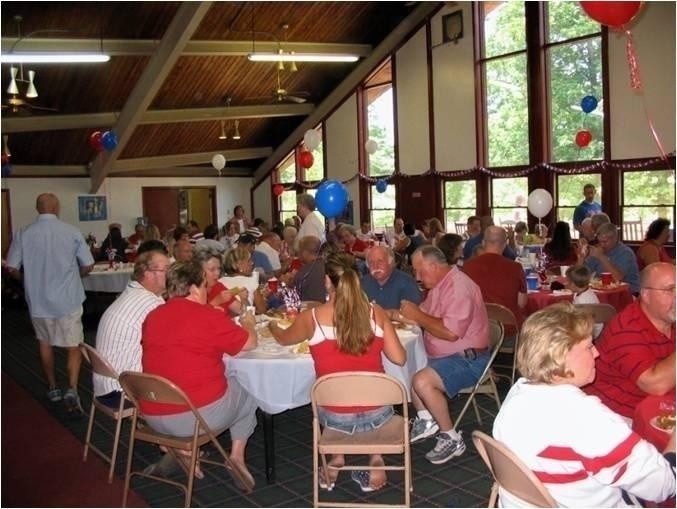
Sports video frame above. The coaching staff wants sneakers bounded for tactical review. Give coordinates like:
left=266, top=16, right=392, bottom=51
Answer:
left=62, top=389, right=85, bottom=419
left=409, top=415, right=439, bottom=444
left=423, top=432, right=466, bottom=465
left=45, top=385, right=62, bottom=403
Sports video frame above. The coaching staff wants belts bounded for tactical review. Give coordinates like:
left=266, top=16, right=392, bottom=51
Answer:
left=460, top=346, right=489, bottom=354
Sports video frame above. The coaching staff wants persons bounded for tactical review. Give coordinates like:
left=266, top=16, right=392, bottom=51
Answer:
left=5, top=190, right=96, bottom=418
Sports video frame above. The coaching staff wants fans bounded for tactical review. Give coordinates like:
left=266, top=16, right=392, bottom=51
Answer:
left=243, top=59, right=310, bottom=106
left=1, top=16, right=59, bottom=118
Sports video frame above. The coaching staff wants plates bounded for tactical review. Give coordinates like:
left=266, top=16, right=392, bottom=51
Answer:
left=591, top=281, right=623, bottom=289
left=647, top=413, right=675, bottom=437
left=254, top=310, right=307, bottom=356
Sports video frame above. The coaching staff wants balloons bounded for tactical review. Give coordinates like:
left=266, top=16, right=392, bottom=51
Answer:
left=304, top=129, right=321, bottom=150
left=365, top=140, right=378, bottom=154
left=2, top=152, right=11, bottom=166
left=581, top=95, right=598, bottom=114
left=579, top=2, right=646, bottom=28
left=575, top=129, right=592, bottom=147
left=101, top=130, right=119, bottom=151
left=89, top=131, right=105, bottom=152
left=2, top=163, right=13, bottom=177
left=299, top=152, right=314, bottom=169
left=212, top=153, right=226, bottom=171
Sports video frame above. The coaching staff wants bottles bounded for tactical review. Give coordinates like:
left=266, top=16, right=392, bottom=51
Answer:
left=369, top=232, right=397, bottom=249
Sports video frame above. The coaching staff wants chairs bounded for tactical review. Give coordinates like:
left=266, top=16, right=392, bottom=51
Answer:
left=75, top=244, right=648, bottom=390
left=78, top=343, right=168, bottom=484
left=311, top=371, right=414, bottom=507
left=119, top=372, right=254, bottom=508
left=469, top=430, right=557, bottom=508
left=446, top=317, right=505, bottom=431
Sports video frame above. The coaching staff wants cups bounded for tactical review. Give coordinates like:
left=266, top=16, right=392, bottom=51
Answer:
left=267, top=278, right=278, bottom=293
left=95, top=262, right=136, bottom=270
left=601, top=271, right=612, bottom=286
left=246, top=305, right=256, bottom=314
left=514, top=246, right=569, bottom=295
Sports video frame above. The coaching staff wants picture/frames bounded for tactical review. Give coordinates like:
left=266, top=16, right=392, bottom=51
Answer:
left=74, top=192, right=110, bottom=223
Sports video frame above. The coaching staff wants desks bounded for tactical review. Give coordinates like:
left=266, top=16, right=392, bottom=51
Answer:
left=223, top=314, right=428, bottom=480
left=631, top=385, right=676, bottom=508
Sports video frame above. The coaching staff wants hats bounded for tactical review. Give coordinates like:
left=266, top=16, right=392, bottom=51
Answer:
left=234, top=233, right=257, bottom=244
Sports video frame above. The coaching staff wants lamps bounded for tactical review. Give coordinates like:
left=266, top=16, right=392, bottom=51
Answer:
left=277, top=48, right=300, bottom=74
left=216, top=118, right=242, bottom=142
left=1, top=134, right=13, bottom=158
left=5, top=64, right=38, bottom=99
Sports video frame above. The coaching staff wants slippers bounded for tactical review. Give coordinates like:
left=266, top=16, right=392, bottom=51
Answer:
left=172, top=444, right=205, bottom=480
left=318, top=477, right=335, bottom=492
left=350, top=471, right=376, bottom=493
left=224, top=455, right=255, bottom=490
